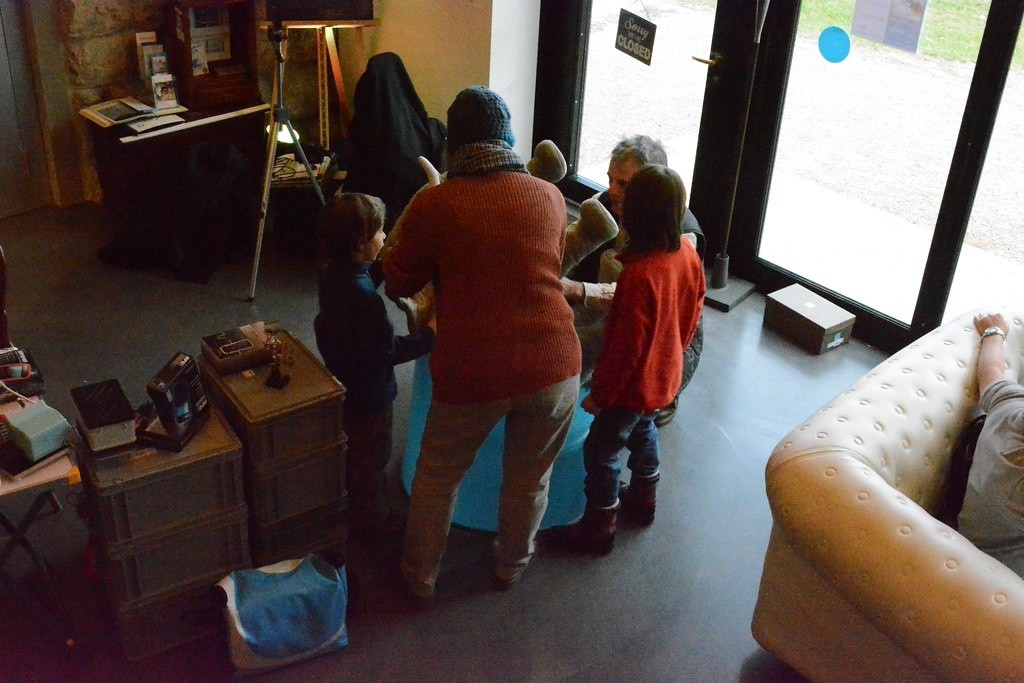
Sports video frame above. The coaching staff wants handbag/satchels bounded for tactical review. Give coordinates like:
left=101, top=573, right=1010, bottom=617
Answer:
left=212, top=543, right=350, bottom=678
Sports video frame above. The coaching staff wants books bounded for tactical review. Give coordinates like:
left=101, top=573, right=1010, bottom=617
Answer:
left=79, top=97, right=159, bottom=127
left=0, top=441, right=70, bottom=481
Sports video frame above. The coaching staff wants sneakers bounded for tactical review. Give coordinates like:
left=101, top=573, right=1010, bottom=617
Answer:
left=494, top=536, right=543, bottom=590
left=382, top=548, right=439, bottom=613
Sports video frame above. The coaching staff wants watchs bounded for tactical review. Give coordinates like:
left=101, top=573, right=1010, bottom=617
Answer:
left=980, top=326, right=1006, bottom=343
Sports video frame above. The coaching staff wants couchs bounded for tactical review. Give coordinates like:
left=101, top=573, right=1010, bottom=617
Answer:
left=752, top=307, right=1023, bottom=682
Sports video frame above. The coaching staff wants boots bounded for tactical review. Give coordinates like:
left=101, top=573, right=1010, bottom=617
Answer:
left=540, top=500, right=620, bottom=554
left=616, top=478, right=658, bottom=526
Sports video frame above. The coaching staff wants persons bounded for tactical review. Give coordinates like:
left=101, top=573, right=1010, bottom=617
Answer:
left=561, top=135, right=706, bottom=426
left=544, top=164, right=706, bottom=556
left=381, top=86, right=581, bottom=601
left=950, top=310, right=1024, bottom=582
left=312, top=192, right=437, bottom=518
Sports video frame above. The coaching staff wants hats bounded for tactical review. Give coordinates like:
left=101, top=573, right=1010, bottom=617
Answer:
left=441, top=85, right=515, bottom=150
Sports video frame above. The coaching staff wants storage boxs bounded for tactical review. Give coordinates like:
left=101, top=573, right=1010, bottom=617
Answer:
left=763, top=282, right=857, bottom=355
left=82, top=397, right=237, bottom=662
left=202, top=325, right=359, bottom=566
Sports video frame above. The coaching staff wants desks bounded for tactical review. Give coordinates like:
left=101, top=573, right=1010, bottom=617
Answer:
left=0, top=452, right=83, bottom=615
